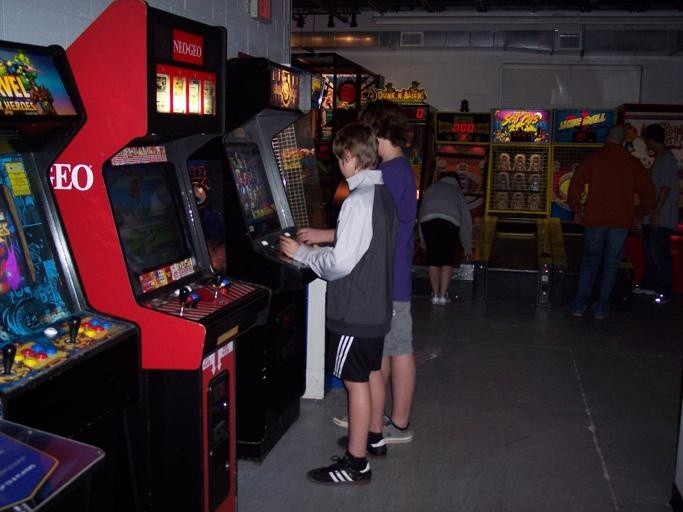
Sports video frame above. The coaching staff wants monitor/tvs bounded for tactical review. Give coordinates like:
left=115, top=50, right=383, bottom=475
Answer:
left=0, top=182, right=35, bottom=298
left=231, top=150, right=276, bottom=222
left=104, top=160, right=189, bottom=274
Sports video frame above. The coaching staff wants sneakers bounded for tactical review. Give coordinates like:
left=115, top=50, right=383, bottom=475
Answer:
left=567, top=304, right=583, bottom=317
left=594, top=311, right=610, bottom=320
left=382, top=417, right=415, bottom=444
left=333, top=411, right=390, bottom=428
left=337, top=435, right=387, bottom=459
left=307, top=454, right=372, bottom=486
left=431, top=291, right=441, bottom=304
left=631, top=287, right=657, bottom=295
left=439, top=292, right=452, bottom=306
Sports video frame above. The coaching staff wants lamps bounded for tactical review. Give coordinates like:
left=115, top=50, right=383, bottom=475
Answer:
left=292, top=11, right=361, bottom=28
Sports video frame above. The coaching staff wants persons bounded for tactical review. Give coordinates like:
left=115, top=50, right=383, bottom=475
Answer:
left=633, top=123, right=679, bottom=307
left=276, top=119, right=387, bottom=485
left=567, top=124, right=657, bottom=320
left=330, top=98, right=416, bottom=445
left=417, top=170, right=474, bottom=306
